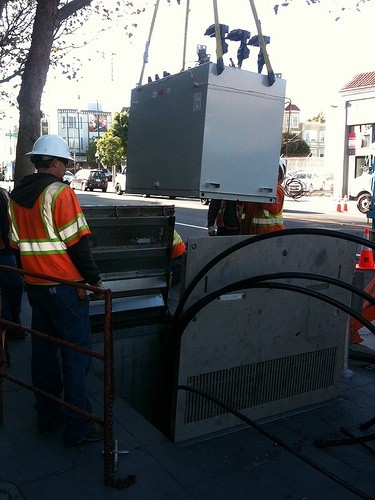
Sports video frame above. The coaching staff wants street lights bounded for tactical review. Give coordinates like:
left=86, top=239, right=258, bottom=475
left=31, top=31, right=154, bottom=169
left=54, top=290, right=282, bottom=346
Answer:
left=73, top=109, right=81, bottom=174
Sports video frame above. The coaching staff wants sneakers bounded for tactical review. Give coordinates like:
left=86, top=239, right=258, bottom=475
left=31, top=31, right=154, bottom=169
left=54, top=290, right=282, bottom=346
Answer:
left=68, top=423, right=104, bottom=441
left=38, top=403, right=59, bottom=427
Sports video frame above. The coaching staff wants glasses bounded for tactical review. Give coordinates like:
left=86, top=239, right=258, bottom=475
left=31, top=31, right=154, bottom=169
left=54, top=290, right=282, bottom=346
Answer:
left=53, top=157, right=68, bottom=167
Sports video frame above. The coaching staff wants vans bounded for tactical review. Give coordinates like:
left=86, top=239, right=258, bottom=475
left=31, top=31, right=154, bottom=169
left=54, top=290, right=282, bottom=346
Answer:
left=348, top=167, right=371, bottom=214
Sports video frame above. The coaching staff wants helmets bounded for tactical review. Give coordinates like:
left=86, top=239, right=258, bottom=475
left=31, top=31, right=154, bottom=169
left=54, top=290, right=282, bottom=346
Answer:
left=279, top=159, right=286, bottom=175
left=25, top=134, right=75, bottom=162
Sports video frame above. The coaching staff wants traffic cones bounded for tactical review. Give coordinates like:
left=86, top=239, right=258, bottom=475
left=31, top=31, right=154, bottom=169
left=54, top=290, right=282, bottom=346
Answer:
left=355, top=226, right=375, bottom=270
left=336, top=195, right=343, bottom=213
left=343, top=195, right=350, bottom=212
left=350, top=331, right=363, bottom=343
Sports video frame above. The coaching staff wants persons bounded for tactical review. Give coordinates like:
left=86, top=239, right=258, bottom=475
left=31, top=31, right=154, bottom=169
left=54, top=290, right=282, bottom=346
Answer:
left=206, top=198, right=248, bottom=237
left=6, top=133, right=106, bottom=449
left=0, top=186, right=29, bottom=343
left=242, top=159, right=287, bottom=235
left=172, top=229, right=191, bottom=285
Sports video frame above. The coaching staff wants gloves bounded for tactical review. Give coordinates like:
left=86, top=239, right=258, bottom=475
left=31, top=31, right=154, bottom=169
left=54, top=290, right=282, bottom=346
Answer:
left=208, top=226, right=215, bottom=236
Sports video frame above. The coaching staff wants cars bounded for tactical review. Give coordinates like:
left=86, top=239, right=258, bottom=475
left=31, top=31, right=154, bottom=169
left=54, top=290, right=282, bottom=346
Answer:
left=114, top=166, right=126, bottom=195
left=70, top=169, right=108, bottom=192
left=102, top=169, right=112, bottom=181
left=63, top=171, right=75, bottom=183
left=294, top=171, right=327, bottom=193
